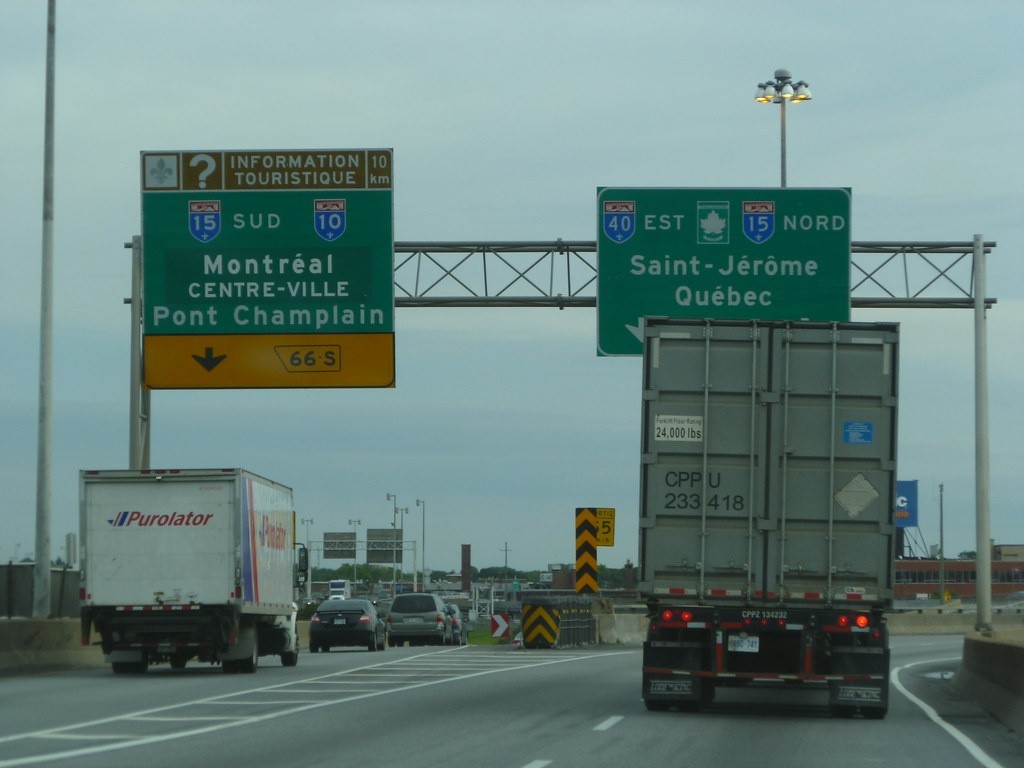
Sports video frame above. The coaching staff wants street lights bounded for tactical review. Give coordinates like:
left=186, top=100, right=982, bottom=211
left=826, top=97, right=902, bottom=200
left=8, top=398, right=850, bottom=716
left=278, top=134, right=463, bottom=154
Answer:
left=536, top=582, right=549, bottom=598
left=416, top=499, right=425, bottom=592
left=386, top=494, right=396, bottom=597
left=349, top=519, right=361, bottom=597
left=301, top=518, right=314, bottom=601
left=752, top=71, right=812, bottom=190
left=396, top=507, right=409, bottom=593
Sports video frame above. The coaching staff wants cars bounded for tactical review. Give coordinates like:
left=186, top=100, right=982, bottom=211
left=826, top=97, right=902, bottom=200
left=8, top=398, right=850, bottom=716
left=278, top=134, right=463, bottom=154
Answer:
left=445, top=603, right=469, bottom=646
left=313, top=593, right=325, bottom=601
left=307, top=599, right=386, bottom=652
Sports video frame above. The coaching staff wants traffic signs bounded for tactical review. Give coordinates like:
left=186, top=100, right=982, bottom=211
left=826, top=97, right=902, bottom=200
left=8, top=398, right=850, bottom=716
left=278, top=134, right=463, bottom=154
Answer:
left=597, top=187, right=850, bottom=353
left=141, top=147, right=395, bottom=387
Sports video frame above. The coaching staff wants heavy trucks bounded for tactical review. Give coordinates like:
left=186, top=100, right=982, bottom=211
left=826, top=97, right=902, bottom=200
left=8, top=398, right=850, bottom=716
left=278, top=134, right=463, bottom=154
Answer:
left=329, top=580, right=351, bottom=600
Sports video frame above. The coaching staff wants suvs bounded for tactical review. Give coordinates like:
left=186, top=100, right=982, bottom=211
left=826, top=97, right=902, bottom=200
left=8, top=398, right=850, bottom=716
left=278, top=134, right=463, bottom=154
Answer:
left=385, top=593, right=454, bottom=645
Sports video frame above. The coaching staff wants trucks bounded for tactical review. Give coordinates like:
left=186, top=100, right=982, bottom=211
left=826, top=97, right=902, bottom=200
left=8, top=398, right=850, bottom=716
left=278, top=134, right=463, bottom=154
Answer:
left=77, top=468, right=310, bottom=672
left=377, top=588, right=391, bottom=601
left=639, top=314, right=899, bottom=718
